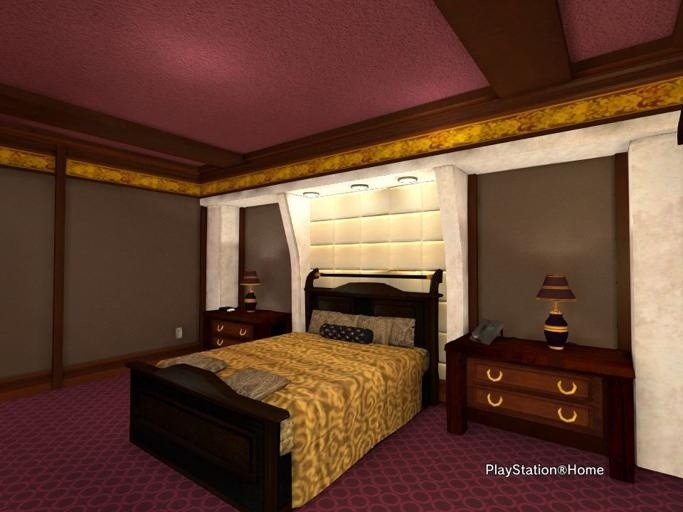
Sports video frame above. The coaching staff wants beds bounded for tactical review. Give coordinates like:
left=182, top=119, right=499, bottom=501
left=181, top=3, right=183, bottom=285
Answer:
left=123, top=267, right=444, bottom=511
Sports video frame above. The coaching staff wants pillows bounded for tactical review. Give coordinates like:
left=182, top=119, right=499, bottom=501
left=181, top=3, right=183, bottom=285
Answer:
left=152, top=352, right=291, bottom=401
left=307, top=308, right=415, bottom=349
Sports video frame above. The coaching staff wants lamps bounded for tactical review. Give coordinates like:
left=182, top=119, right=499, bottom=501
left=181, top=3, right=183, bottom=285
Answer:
left=238, top=269, right=261, bottom=313
left=535, top=271, right=575, bottom=351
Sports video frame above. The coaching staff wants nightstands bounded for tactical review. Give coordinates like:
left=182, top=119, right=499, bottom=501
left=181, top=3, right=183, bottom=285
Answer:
left=203, top=306, right=290, bottom=350
left=442, top=334, right=635, bottom=486
